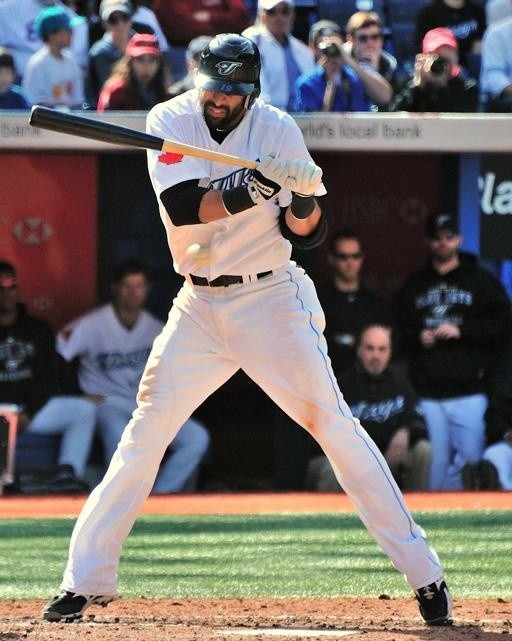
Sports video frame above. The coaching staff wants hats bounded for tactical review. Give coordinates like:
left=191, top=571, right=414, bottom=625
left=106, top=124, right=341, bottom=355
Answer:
left=126, top=34, right=160, bottom=58
left=100, top=0, right=134, bottom=22
left=432, top=213, right=458, bottom=237
left=34, top=6, right=88, bottom=32
left=423, top=27, right=458, bottom=52
left=260, top=0, right=293, bottom=11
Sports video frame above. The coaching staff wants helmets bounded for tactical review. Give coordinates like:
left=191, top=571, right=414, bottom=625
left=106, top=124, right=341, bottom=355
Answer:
left=197, top=33, right=260, bottom=109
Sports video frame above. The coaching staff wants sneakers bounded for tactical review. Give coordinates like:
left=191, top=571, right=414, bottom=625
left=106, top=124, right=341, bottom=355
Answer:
left=42, top=590, right=119, bottom=623
left=414, top=577, right=451, bottom=624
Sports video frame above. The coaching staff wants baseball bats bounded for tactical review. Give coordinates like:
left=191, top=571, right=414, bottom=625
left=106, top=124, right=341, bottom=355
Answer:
left=27, top=106, right=320, bottom=193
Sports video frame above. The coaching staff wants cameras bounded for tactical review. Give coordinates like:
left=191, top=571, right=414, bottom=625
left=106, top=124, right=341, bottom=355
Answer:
left=321, top=41, right=339, bottom=60
left=429, top=58, right=449, bottom=78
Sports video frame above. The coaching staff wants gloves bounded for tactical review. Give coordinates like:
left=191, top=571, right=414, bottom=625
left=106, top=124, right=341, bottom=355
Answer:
left=249, top=152, right=288, bottom=203
left=289, top=158, right=322, bottom=197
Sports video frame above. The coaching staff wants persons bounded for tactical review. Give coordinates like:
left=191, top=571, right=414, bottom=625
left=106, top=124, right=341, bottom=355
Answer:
left=305, top=314, right=431, bottom=493
left=316, top=228, right=390, bottom=373
left=0, top=259, right=96, bottom=498
left=395, top=208, right=511, bottom=492
left=42, top=33, right=452, bottom=627
left=54, top=254, right=212, bottom=495
left=0, top=0, right=512, bottom=113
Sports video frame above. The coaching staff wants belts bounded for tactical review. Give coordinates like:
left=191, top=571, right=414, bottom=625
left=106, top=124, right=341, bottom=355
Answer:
left=190, top=271, right=272, bottom=287
